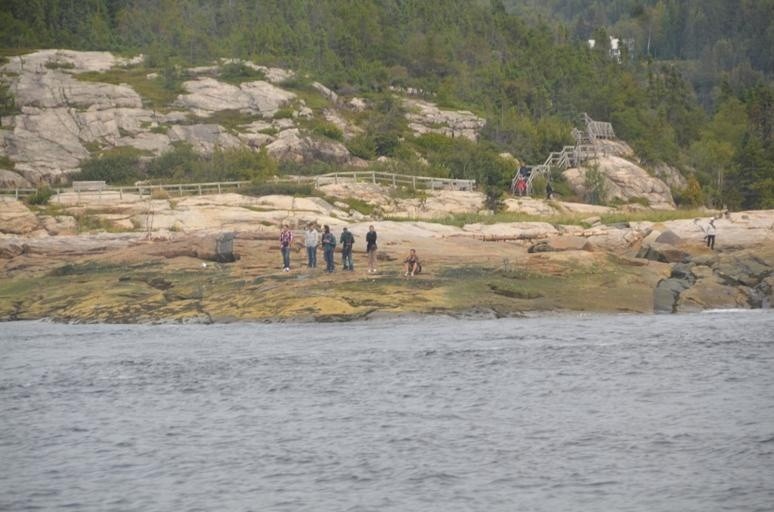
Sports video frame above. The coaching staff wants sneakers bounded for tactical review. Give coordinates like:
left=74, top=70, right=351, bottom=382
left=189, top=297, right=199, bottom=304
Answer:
left=368, top=268, right=377, bottom=274
left=404, top=272, right=415, bottom=277
left=283, top=268, right=290, bottom=272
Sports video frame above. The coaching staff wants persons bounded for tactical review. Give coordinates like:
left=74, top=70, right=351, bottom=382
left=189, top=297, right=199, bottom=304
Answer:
left=278, top=224, right=292, bottom=272
left=706, top=218, right=715, bottom=251
left=339, top=227, right=354, bottom=272
left=303, top=224, right=318, bottom=268
left=402, top=249, right=422, bottom=276
left=320, top=225, right=335, bottom=273
left=520, top=162, right=527, bottom=176
left=516, top=177, right=526, bottom=196
left=546, top=183, right=552, bottom=197
left=365, top=224, right=379, bottom=274
